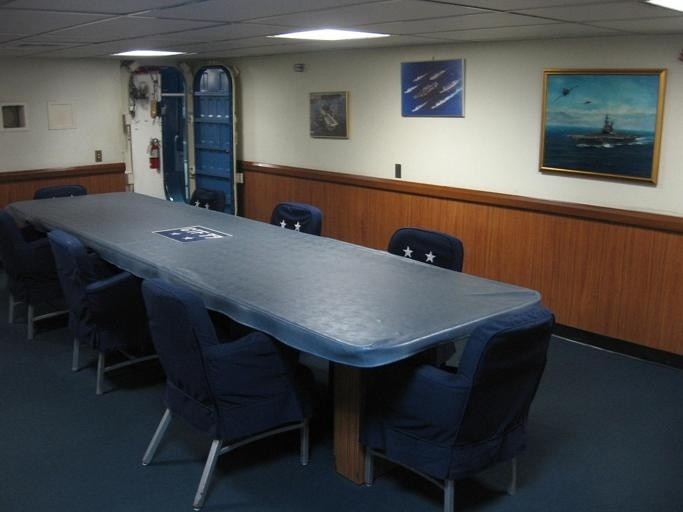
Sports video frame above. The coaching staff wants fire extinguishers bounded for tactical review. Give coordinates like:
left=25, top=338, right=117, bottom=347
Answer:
left=146, top=138, right=160, bottom=169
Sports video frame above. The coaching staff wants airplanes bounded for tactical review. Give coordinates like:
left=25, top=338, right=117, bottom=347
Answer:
left=554, top=83, right=578, bottom=103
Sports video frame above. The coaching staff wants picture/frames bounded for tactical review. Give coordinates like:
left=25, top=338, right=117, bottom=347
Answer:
left=310, top=91, right=349, bottom=139
left=48, top=100, right=76, bottom=130
left=538, top=67, right=668, bottom=185
left=0, top=102, right=30, bottom=132
left=401, top=57, right=466, bottom=117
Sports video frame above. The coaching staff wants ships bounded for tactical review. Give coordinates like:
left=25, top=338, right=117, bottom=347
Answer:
left=568, top=113, right=640, bottom=145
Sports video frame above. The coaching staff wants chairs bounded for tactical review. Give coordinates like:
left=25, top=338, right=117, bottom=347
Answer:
left=34, top=186, right=88, bottom=200
left=1, top=205, right=67, bottom=344
left=269, top=202, right=323, bottom=237
left=188, top=185, right=226, bottom=215
left=139, top=278, right=319, bottom=512
left=47, top=230, right=158, bottom=396
left=329, top=304, right=553, bottom=512
left=386, top=227, right=465, bottom=365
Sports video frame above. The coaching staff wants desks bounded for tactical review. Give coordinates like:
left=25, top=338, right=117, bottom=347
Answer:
left=10, top=189, right=540, bottom=486
